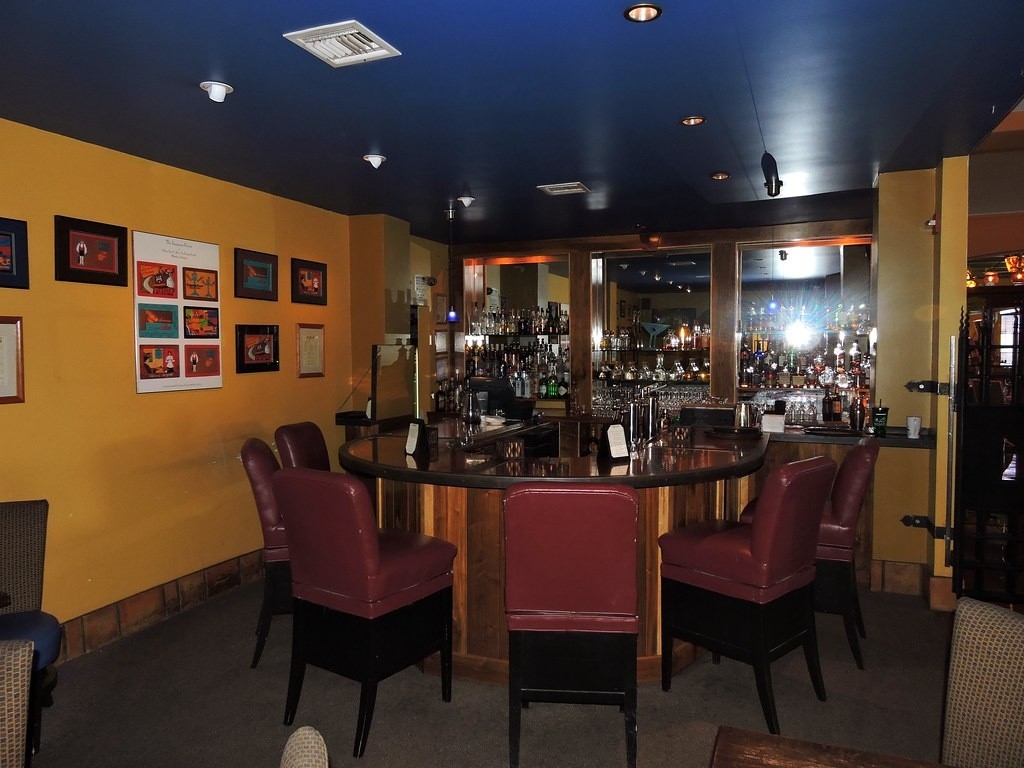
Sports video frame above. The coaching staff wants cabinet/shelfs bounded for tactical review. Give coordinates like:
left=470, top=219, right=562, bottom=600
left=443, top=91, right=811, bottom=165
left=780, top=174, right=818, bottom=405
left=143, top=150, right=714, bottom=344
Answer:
left=468, top=329, right=857, bottom=424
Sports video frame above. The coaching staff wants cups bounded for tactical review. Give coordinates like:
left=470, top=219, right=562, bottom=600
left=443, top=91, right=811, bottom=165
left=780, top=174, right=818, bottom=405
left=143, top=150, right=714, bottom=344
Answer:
left=460, top=427, right=474, bottom=446
left=872, top=399, right=889, bottom=438
left=907, top=416, right=922, bottom=439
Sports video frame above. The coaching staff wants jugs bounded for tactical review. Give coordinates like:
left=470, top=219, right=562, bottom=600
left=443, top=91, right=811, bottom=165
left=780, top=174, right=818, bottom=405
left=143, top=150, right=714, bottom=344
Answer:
left=461, top=388, right=481, bottom=424
left=734, top=401, right=753, bottom=428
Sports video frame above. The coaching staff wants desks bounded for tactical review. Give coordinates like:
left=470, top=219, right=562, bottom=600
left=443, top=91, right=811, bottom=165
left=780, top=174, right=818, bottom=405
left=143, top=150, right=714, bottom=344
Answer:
left=336, top=424, right=769, bottom=688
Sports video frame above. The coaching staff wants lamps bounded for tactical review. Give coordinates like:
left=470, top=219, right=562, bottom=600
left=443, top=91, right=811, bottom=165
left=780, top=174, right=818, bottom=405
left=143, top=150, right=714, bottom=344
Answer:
left=763, top=176, right=784, bottom=197
left=456, top=196, right=475, bottom=208
left=363, top=155, right=386, bottom=169
left=779, top=250, right=788, bottom=261
left=200, top=81, right=235, bottom=103
left=619, top=264, right=691, bottom=293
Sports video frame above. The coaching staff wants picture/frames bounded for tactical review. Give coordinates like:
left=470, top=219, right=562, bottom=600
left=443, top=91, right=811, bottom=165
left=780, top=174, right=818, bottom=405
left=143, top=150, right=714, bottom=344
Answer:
left=235, top=324, right=279, bottom=373
left=233, top=247, right=278, bottom=301
left=54, top=215, right=128, bottom=287
left=290, top=258, right=327, bottom=305
left=0, top=217, right=29, bottom=290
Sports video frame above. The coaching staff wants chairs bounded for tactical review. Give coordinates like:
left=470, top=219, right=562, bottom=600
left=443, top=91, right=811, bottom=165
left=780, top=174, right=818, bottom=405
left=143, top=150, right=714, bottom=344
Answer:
left=269, top=468, right=456, bottom=760
left=658, top=459, right=839, bottom=736
left=276, top=421, right=330, bottom=472
left=241, top=438, right=296, bottom=668
left=941, top=597, right=1024, bottom=767
left=0, top=501, right=63, bottom=768
left=738, top=438, right=880, bottom=670
left=499, top=485, right=642, bottom=768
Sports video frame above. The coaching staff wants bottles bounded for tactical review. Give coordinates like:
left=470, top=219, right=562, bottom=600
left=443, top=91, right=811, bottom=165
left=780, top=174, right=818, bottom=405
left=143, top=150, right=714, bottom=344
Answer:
left=435, top=337, right=571, bottom=411
left=737, top=335, right=871, bottom=389
left=757, top=385, right=870, bottom=431
left=447, top=305, right=458, bottom=322
left=470, top=302, right=569, bottom=335
left=746, top=298, right=871, bottom=331
left=598, top=354, right=710, bottom=381
left=591, top=385, right=710, bottom=419
left=599, top=309, right=710, bottom=351
